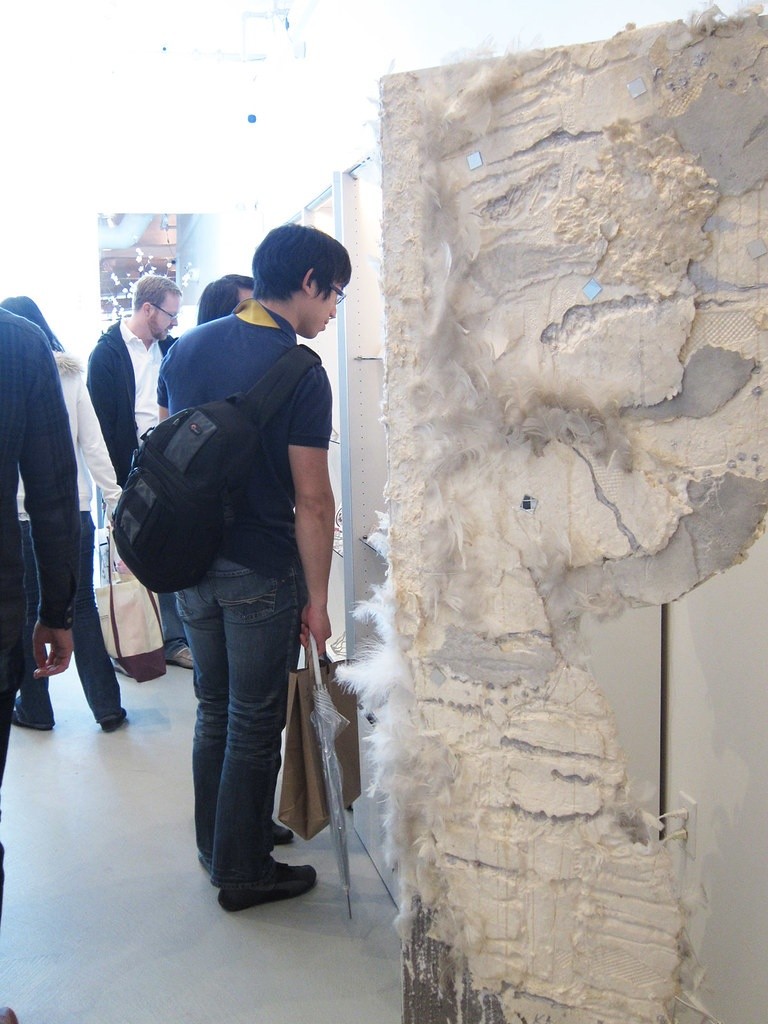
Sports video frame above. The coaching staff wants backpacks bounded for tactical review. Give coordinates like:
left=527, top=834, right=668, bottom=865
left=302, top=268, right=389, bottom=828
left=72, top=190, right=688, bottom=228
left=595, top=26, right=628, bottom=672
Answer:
left=109, top=343, right=321, bottom=595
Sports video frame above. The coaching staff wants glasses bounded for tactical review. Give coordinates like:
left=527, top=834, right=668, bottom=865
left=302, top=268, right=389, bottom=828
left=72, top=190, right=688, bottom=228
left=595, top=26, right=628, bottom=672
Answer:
left=327, top=282, right=347, bottom=304
left=151, top=303, right=178, bottom=321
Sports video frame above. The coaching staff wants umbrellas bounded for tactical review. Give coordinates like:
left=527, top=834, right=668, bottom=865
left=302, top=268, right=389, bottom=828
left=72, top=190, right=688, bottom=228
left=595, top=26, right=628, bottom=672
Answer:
left=302, top=628, right=356, bottom=920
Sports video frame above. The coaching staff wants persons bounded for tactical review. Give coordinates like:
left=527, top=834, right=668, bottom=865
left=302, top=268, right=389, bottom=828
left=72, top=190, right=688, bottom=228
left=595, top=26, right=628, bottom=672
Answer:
left=0, top=295, right=126, bottom=733
left=85, top=276, right=195, bottom=670
left=0, top=299, right=81, bottom=1023
left=154, top=221, right=352, bottom=912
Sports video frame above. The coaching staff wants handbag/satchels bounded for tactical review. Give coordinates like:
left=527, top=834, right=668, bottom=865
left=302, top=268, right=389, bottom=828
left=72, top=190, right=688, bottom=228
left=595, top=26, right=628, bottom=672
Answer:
left=95, top=571, right=167, bottom=683
left=278, top=635, right=362, bottom=841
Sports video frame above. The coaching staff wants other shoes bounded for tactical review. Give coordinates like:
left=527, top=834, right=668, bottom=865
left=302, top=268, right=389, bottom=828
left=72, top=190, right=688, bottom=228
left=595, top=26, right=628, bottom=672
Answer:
left=109, top=656, right=135, bottom=678
left=100, top=707, right=127, bottom=732
left=167, top=644, right=194, bottom=670
left=11, top=710, right=54, bottom=731
